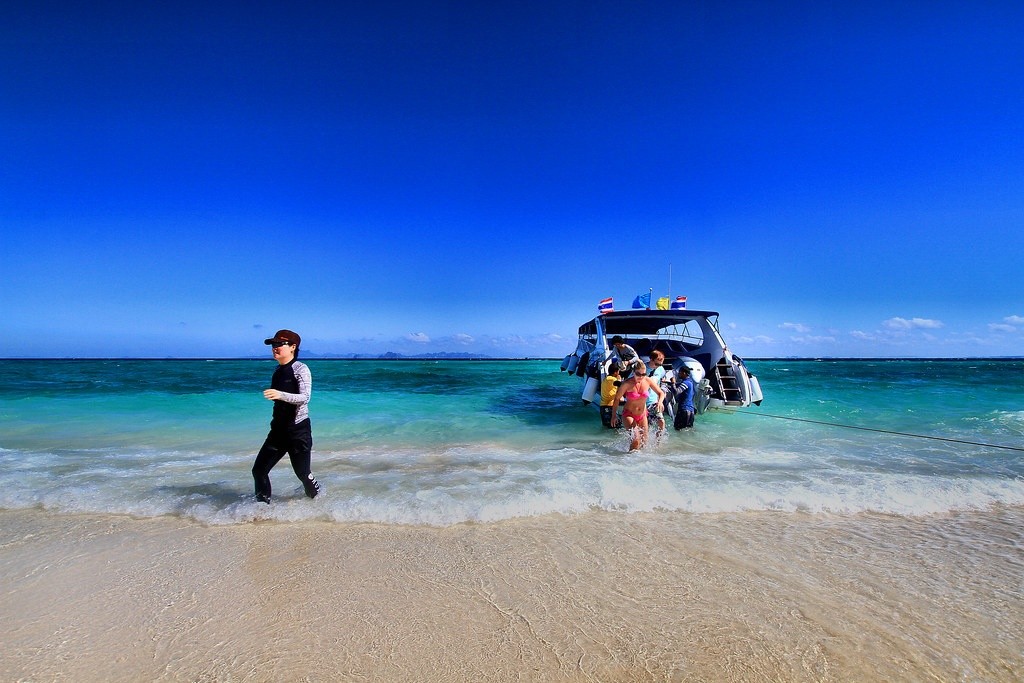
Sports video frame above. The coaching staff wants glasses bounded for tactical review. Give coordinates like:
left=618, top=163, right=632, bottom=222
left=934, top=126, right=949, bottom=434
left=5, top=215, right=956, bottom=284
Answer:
left=635, top=372, right=646, bottom=376
left=272, top=342, right=289, bottom=348
left=654, top=363, right=663, bottom=366
left=613, top=344, right=620, bottom=347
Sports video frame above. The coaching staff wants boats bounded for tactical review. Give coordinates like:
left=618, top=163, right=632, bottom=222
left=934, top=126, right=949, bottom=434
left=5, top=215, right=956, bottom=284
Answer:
left=576, top=308, right=763, bottom=415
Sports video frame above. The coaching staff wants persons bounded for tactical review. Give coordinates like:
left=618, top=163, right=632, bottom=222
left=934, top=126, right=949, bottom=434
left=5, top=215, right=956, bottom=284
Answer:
left=600, top=336, right=694, bottom=454
left=251, top=329, right=321, bottom=504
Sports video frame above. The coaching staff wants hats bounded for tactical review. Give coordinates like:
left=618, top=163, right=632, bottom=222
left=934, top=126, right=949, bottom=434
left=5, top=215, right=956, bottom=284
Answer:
left=264, top=330, right=300, bottom=345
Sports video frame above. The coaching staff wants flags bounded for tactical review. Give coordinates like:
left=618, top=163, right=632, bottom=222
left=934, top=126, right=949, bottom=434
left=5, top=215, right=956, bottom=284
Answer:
left=676, top=296, right=686, bottom=300
left=656, top=298, right=670, bottom=310
left=598, top=297, right=615, bottom=314
left=671, top=302, right=685, bottom=309
left=632, top=293, right=651, bottom=309
